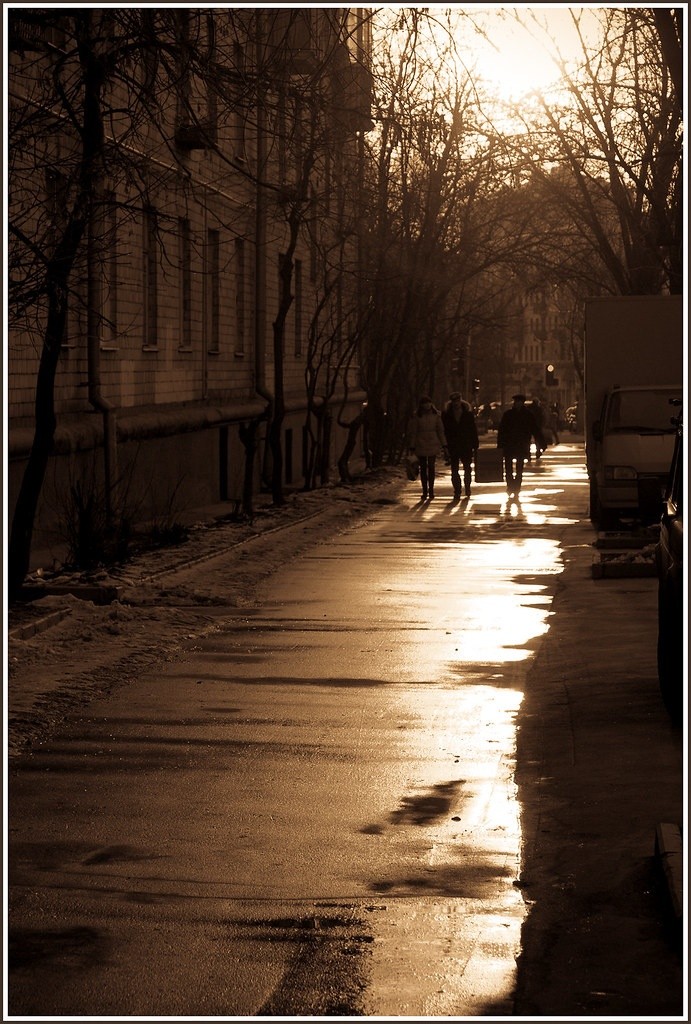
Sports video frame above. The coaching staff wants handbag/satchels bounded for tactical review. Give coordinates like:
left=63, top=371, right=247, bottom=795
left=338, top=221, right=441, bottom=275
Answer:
left=405, top=448, right=419, bottom=481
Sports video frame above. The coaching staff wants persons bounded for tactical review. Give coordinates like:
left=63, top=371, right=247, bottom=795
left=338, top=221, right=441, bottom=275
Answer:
left=405, top=392, right=479, bottom=500
left=497, top=393, right=567, bottom=489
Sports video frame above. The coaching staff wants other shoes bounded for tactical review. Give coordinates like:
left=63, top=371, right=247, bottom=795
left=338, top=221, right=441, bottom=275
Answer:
left=454, top=495, right=460, bottom=500
left=429, top=493, right=435, bottom=498
left=466, top=491, right=471, bottom=497
left=421, top=492, right=428, bottom=498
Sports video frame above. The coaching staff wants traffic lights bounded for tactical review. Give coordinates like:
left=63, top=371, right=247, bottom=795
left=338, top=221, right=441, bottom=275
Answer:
left=472, top=376, right=481, bottom=394
left=546, top=364, right=555, bottom=386
left=450, top=357, right=462, bottom=376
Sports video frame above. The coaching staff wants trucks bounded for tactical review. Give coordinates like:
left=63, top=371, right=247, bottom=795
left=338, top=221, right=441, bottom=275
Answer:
left=582, top=295, right=683, bottom=527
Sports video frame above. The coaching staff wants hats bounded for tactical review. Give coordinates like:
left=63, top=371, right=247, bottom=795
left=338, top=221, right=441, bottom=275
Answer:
left=449, top=391, right=462, bottom=400
left=512, top=394, right=526, bottom=402
left=420, top=395, right=432, bottom=403
